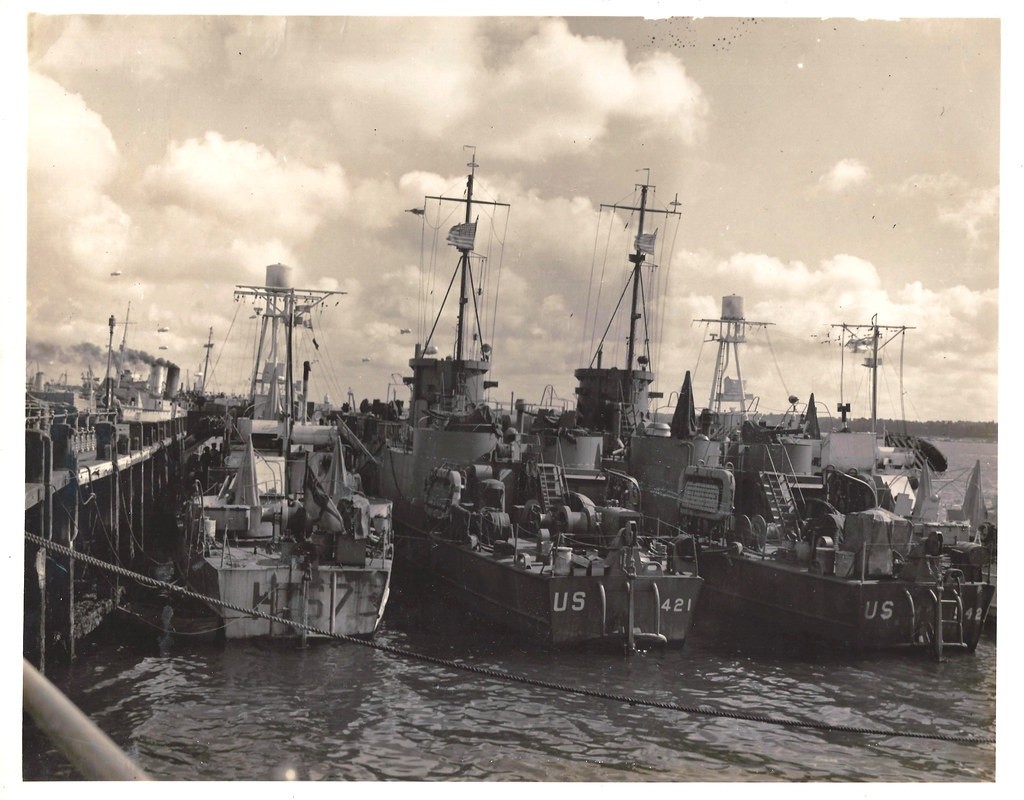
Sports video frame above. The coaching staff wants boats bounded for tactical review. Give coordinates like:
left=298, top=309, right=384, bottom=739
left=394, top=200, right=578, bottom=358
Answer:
left=160, top=144, right=995, bottom=657
